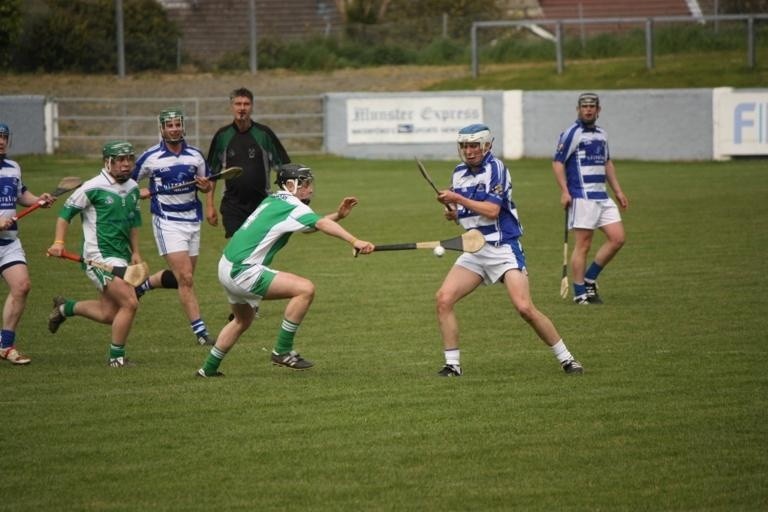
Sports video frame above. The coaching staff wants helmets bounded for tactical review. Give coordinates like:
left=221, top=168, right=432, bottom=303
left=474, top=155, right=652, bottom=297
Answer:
left=579, top=93, right=599, bottom=125
left=0, top=122, right=12, bottom=154
left=160, top=107, right=186, bottom=144
left=457, top=124, right=494, bottom=166
left=103, top=139, right=137, bottom=182
left=276, top=164, right=312, bottom=194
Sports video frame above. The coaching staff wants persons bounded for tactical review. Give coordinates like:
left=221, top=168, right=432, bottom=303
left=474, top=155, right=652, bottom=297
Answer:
left=44, top=139, right=150, bottom=372
left=206, top=87, right=292, bottom=326
left=550, top=92, right=630, bottom=305
left=127, top=106, right=219, bottom=347
left=431, top=122, right=586, bottom=376
left=194, top=161, right=376, bottom=383
left=0, top=122, right=61, bottom=365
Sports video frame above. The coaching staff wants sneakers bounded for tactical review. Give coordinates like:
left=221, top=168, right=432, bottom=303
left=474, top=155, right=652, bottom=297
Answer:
left=0, top=346, right=31, bottom=366
left=135, top=284, right=144, bottom=302
left=563, top=360, right=584, bottom=374
left=436, top=364, right=464, bottom=377
left=271, top=349, right=314, bottom=370
left=583, top=278, right=602, bottom=305
left=48, top=295, right=66, bottom=334
left=197, top=369, right=226, bottom=377
left=108, top=357, right=136, bottom=368
left=573, top=294, right=593, bottom=305
left=197, top=332, right=217, bottom=345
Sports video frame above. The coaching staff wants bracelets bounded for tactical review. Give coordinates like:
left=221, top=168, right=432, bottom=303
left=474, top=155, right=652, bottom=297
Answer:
left=53, top=239, right=65, bottom=246
left=349, top=237, right=358, bottom=247
